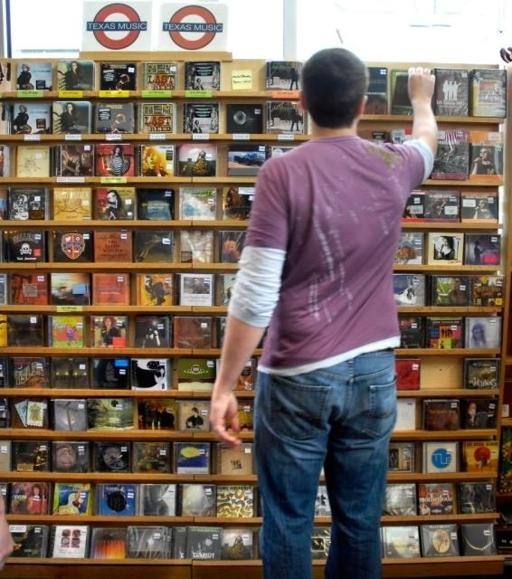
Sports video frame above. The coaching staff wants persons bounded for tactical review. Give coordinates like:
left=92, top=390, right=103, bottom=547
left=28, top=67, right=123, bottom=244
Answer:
left=191, top=118, right=203, bottom=133
left=288, top=63, right=299, bottom=91
left=472, top=323, right=493, bottom=349
left=439, top=237, right=455, bottom=262
left=465, top=400, right=488, bottom=429
left=105, top=191, right=131, bottom=220
left=441, top=77, right=461, bottom=103
left=12, top=105, right=30, bottom=134
left=186, top=408, right=205, bottom=431
left=472, top=239, right=485, bottom=263
left=99, top=318, right=121, bottom=348
left=18, top=62, right=36, bottom=90
left=141, top=324, right=160, bottom=349
left=26, top=485, right=46, bottom=514
left=61, top=60, right=89, bottom=90
left=289, top=103, right=301, bottom=132
left=450, top=279, right=470, bottom=306
left=195, top=77, right=208, bottom=90
left=210, top=48, right=439, bottom=578
left=472, top=147, right=495, bottom=177
left=103, top=146, right=126, bottom=176
left=473, top=198, right=493, bottom=220
left=58, top=104, right=78, bottom=130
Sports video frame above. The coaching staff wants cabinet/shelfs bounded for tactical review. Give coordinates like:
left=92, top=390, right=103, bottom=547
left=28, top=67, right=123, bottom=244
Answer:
left=0, top=50, right=512, bottom=579
